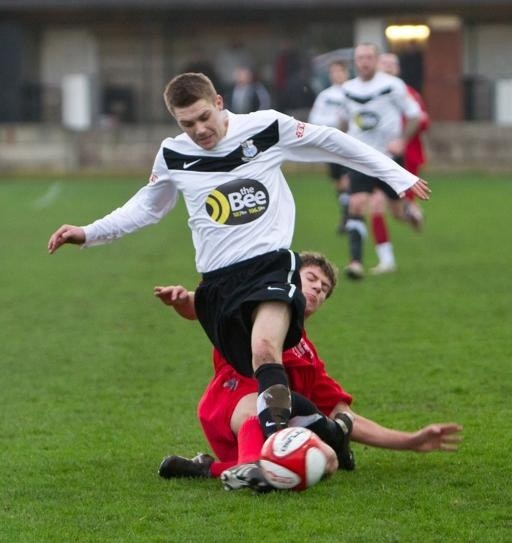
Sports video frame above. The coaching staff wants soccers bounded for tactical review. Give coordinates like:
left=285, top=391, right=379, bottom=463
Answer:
left=260, top=427, right=325, bottom=491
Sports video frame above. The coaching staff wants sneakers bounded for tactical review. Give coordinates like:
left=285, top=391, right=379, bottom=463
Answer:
left=334, top=412, right=355, bottom=469
left=221, top=463, right=273, bottom=491
left=158, top=453, right=215, bottom=477
left=346, top=260, right=396, bottom=278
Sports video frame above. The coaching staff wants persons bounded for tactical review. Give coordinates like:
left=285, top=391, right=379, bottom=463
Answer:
left=56, top=57, right=94, bottom=170
left=306, top=58, right=351, bottom=232
left=222, top=63, right=274, bottom=112
left=47, top=69, right=432, bottom=492
left=335, top=39, right=424, bottom=278
left=367, top=47, right=429, bottom=273
left=153, top=252, right=463, bottom=478
